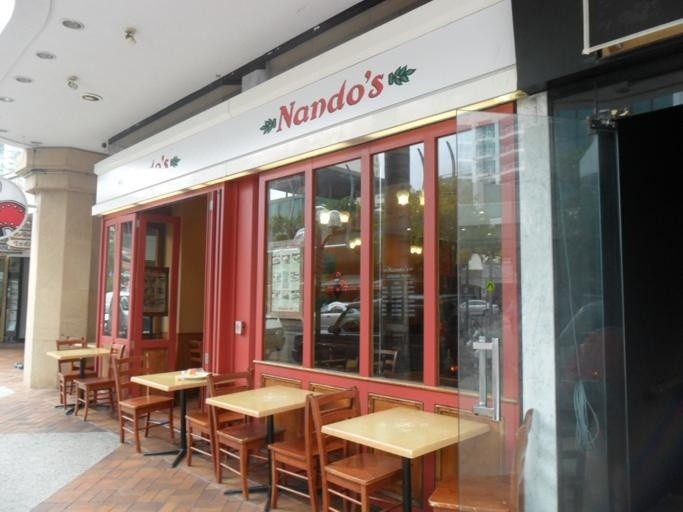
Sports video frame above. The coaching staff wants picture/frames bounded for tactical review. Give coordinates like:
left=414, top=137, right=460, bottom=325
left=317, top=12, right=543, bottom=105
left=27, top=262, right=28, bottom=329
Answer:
left=264, top=240, right=304, bottom=320
left=143, top=265, right=169, bottom=317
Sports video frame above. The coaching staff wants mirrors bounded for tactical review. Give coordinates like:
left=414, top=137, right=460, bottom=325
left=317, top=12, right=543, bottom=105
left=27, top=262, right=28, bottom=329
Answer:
left=0, top=175, right=29, bottom=241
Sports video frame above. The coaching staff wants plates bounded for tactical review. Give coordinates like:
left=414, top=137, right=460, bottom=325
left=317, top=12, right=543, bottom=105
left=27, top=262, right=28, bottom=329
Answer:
left=178, top=370, right=210, bottom=379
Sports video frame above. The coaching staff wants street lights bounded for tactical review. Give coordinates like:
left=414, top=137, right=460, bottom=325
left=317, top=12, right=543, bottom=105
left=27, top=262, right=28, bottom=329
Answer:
left=314, top=207, right=350, bottom=368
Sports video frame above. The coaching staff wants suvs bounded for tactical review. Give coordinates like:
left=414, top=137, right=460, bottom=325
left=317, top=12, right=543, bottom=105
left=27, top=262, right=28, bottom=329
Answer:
left=293, top=290, right=449, bottom=374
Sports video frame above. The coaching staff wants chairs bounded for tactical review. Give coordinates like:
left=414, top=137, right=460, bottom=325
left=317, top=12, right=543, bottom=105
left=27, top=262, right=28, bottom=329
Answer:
left=309, top=386, right=403, bottom=512
left=188, top=340, right=202, bottom=368
left=316, top=339, right=401, bottom=376
left=428, top=408, right=534, bottom=511
left=267, top=385, right=358, bottom=512
left=185, top=368, right=255, bottom=477
left=57, top=335, right=87, bottom=410
left=207, top=369, right=287, bottom=499
left=74, top=343, right=127, bottom=421
left=111, top=351, right=174, bottom=453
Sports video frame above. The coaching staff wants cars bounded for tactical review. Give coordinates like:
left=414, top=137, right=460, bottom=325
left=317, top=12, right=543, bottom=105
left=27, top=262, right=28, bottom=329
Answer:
left=261, top=315, right=286, bottom=359
left=312, top=300, right=362, bottom=330
left=460, top=300, right=500, bottom=317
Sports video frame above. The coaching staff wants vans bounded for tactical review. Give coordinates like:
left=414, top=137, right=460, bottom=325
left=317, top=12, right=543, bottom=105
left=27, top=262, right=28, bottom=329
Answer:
left=102, top=291, right=153, bottom=335
